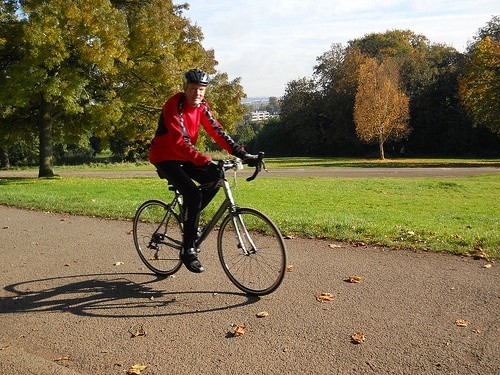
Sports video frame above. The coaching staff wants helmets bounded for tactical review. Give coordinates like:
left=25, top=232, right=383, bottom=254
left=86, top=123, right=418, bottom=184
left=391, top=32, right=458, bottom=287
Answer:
left=184, top=68, right=211, bottom=86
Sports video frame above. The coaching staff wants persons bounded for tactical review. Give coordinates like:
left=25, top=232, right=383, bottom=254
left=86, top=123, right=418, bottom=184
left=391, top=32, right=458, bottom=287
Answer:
left=148, top=67, right=258, bottom=273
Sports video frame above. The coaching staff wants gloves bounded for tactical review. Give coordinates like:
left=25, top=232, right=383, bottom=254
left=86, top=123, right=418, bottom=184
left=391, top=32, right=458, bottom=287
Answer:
left=204, top=158, right=224, bottom=174
left=241, top=154, right=263, bottom=167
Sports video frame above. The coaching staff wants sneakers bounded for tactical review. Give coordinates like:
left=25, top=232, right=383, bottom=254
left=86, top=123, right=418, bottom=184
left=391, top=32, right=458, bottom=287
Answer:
left=179, top=243, right=204, bottom=273
left=195, top=227, right=203, bottom=238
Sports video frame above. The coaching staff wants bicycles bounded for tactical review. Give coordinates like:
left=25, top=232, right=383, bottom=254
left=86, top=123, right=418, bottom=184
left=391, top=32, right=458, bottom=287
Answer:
left=133, top=152, right=286, bottom=296
